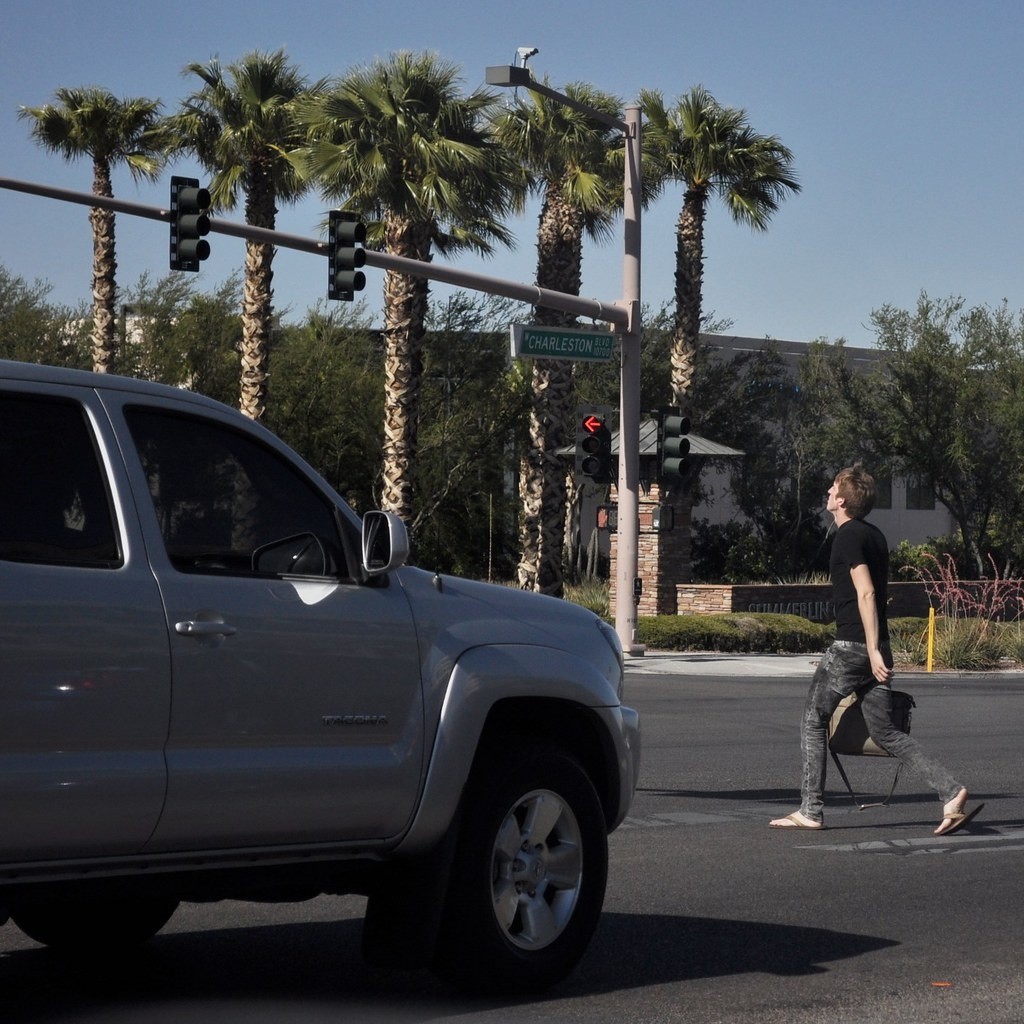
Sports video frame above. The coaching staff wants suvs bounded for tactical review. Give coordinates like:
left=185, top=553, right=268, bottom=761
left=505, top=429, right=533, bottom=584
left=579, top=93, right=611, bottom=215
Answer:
left=0, top=361, right=639, bottom=989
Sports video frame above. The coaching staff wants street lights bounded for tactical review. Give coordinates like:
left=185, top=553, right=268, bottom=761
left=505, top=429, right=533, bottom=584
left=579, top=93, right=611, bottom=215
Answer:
left=485, top=67, right=645, bottom=656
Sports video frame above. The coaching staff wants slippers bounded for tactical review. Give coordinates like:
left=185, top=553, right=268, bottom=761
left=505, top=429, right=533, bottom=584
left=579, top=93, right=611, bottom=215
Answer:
left=935, top=802, right=985, bottom=836
left=769, top=815, right=828, bottom=830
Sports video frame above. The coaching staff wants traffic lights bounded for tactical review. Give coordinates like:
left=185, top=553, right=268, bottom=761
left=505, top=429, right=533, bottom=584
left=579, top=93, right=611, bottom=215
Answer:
left=169, top=176, right=212, bottom=274
left=652, top=507, right=675, bottom=532
left=576, top=408, right=609, bottom=485
left=652, top=405, right=692, bottom=487
left=597, top=507, right=619, bottom=529
left=329, top=211, right=367, bottom=300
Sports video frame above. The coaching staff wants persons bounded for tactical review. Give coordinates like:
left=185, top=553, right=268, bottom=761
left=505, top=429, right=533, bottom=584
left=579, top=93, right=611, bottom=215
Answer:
left=769, top=457, right=986, bottom=835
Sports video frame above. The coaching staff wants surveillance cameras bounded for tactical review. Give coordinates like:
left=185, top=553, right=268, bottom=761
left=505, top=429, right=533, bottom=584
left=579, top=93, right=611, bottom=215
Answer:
left=517, top=47, right=540, bottom=56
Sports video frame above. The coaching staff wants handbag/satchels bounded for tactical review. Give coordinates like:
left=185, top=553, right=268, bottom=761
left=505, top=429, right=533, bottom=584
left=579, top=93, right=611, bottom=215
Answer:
left=827, top=687, right=916, bottom=810
